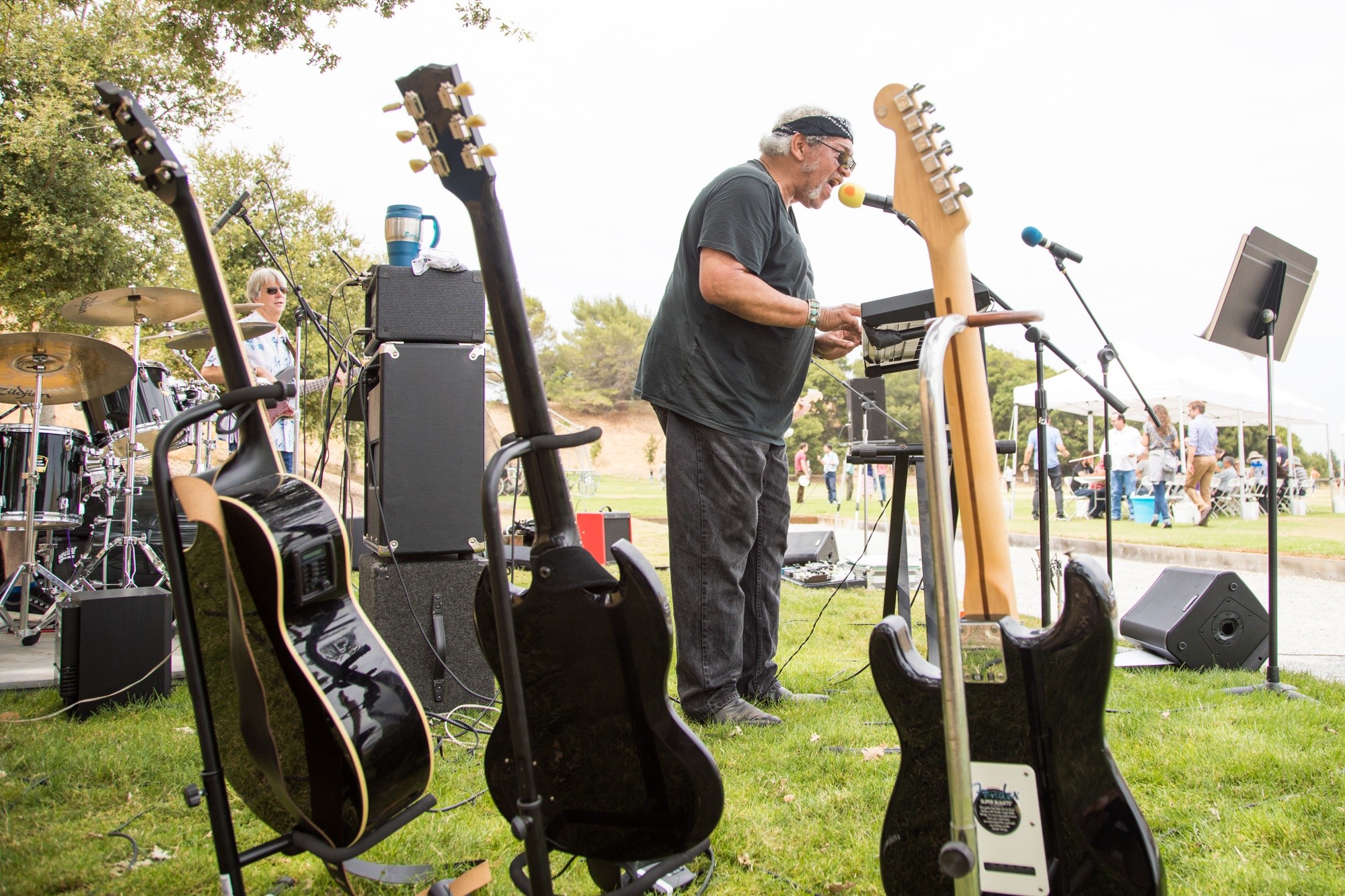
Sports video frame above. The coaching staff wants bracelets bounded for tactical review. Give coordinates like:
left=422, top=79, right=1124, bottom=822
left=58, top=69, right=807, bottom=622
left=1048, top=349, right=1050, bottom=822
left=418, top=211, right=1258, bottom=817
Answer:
left=805, top=296, right=822, bottom=329
left=1023, top=463, right=1030, bottom=466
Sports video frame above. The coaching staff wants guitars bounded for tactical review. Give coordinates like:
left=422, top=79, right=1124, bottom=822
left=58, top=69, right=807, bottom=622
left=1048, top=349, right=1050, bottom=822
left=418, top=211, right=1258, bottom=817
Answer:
left=252, top=365, right=364, bottom=430
left=89, top=72, right=438, bottom=856
left=379, top=61, right=730, bottom=884
left=862, top=80, right=1178, bottom=896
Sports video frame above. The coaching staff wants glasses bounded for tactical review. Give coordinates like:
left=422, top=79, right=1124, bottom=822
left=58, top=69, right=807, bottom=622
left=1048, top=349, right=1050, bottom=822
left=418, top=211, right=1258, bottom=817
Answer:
left=260, top=286, right=288, bottom=295
left=783, top=123, right=857, bottom=171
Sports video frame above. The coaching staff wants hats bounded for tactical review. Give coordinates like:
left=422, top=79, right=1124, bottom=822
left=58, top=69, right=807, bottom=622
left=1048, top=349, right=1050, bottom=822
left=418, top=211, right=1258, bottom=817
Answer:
left=1246, top=451, right=1265, bottom=463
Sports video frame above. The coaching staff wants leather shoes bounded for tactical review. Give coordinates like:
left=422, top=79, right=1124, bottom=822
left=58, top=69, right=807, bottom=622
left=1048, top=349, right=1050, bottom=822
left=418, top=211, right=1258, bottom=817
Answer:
left=761, top=687, right=829, bottom=703
left=701, top=698, right=780, bottom=725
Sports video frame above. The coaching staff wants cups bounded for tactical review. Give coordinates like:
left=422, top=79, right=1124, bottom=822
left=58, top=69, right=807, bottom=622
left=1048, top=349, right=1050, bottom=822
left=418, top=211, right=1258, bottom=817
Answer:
left=385, top=204, right=441, bottom=266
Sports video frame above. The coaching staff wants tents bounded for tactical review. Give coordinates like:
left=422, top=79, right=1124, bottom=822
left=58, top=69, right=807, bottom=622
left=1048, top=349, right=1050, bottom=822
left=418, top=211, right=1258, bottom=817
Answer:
left=1010, top=329, right=1337, bottom=515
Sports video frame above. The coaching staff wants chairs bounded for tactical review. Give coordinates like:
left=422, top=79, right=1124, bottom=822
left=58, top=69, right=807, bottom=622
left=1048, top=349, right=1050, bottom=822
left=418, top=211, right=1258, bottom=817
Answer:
left=1064, top=474, right=1315, bottom=521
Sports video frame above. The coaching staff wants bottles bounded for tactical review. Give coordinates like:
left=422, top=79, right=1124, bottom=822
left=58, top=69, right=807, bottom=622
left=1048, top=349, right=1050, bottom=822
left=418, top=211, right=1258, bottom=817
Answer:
left=1023, top=470, right=1029, bottom=483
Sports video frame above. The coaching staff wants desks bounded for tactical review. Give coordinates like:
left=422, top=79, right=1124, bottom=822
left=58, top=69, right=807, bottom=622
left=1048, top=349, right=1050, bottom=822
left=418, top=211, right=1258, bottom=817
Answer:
left=1074, top=476, right=1106, bottom=520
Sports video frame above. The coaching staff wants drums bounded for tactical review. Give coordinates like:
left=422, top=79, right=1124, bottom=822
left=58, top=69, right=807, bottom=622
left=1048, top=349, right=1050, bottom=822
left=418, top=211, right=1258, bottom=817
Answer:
left=35, top=472, right=198, bottom=625
left=78, top=461, right=124, bottom=500
left=0, top=423, right=88, bottom=532
left=79, top=359, right=190, bottom=461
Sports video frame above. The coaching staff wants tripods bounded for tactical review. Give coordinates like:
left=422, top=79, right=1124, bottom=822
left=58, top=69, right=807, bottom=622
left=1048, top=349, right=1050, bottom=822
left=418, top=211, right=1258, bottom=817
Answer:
left=1193, top=226, right=1329, bottom=712
left=0, top=302, right=171, bottom=646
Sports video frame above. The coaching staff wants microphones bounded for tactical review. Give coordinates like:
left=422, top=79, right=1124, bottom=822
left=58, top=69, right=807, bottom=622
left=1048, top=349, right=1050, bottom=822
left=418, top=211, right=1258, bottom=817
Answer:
left=1021, top=226, right=1084, bottom=263
left=838, top=182, right=894, bottom=212
left=313, top=310, right=329, bottom=321
left=209, top=180, right=261, bottom=235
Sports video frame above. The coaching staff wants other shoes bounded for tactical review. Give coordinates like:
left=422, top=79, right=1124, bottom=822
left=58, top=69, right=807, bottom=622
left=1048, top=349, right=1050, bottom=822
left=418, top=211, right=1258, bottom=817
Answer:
left=1151, top=514, right=1159, bottom=526
left=1195, top=507, right=1213, bottom=526
left=1128, top=515, right=1134, bottom=520
left=1163, top=519, right=1172, bottom=528
left=1056, top=515, right=1068, bottom=520
left=1088, top=514, right=1104, bottom=518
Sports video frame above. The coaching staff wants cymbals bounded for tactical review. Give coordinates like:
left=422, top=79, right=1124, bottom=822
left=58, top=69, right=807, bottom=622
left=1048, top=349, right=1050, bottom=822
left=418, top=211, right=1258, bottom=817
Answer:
left=60, top=286, right=204, bottom=327
left=0, top=331, right=138, bottom=406
left=161, top=302, right=265, bottom=326
left=165, top=322, right=277, bottom=350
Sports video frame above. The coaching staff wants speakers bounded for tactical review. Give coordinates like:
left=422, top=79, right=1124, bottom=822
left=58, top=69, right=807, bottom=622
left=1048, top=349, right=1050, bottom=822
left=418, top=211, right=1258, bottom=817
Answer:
left=781, top=530, right=839, bottom=568
left=577, top=512, right=633, bottom=564
left=845, top=378, right=888, bottom=444
left=357, top=261, right=495, bottom=722
left=1119, top=565, right=1269, bottom=673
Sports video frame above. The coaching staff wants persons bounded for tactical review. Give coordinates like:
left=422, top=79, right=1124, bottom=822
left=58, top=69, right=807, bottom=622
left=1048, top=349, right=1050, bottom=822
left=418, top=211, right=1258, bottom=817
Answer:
left=199, top=267, right=356, bottom=475
left=794, top=440, right=811, bottom=503
left=816, top=443, right=839, bottom=505
left=864, top=461, right=887, bottom=502
left=1003, top=465, right=1014, bottom=491
left=1070, top=401, right=1307, bottom=530
left=633, top=101, right=863, bottom=729
left=843, top=457, right=853, bottom=501
left=1019, top=416, right=1070, bottom=520
left=0, top=402, right=57, bottom=622
